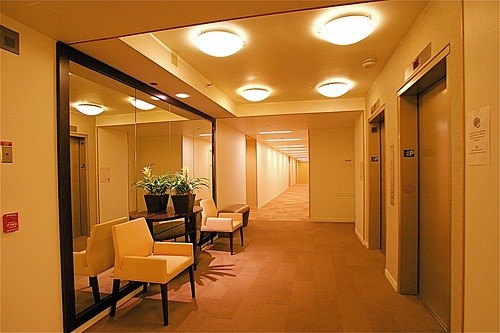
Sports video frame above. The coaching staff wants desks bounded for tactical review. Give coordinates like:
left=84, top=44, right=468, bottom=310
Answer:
left=127, top=207, right=186, bottom=236
left=147, top=206, right=202, bottom=271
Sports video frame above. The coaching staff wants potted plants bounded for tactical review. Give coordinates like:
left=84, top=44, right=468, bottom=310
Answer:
left=130, top=166, right=170, bottom=213
left=170, top=166, right=210, bottom=213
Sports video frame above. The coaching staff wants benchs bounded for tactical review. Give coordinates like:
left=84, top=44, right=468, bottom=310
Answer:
left=217, top=204, right=250, bottom=238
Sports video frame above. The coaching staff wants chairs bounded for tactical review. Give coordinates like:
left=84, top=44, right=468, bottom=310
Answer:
left=73, top=217, right=128, bottom=302
left=199, top=198, right=244, bottom=255
left=109, top=216, right=196, bottom=326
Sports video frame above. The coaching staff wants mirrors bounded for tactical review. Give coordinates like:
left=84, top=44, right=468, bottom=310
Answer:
left=69, top=60, right=213, bottom=315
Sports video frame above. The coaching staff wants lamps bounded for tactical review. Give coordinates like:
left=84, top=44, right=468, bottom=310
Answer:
left=239, top=89, right=271, bottom=103
left=194, top=32, right=244, bottom=58
left=131, top=98, right=156, bottom=110
left=75, top=104, right=104, bottom=116
left=317, top=14, right=376, bottom=45
left=315, top=81, right=352, bottom=97
